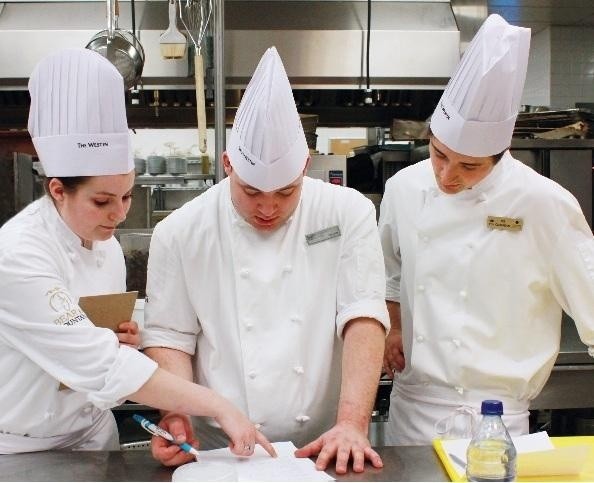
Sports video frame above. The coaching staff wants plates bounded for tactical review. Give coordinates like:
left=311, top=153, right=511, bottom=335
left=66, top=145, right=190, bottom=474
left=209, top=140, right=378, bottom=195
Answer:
left=132, top=155, right=188, bottom=174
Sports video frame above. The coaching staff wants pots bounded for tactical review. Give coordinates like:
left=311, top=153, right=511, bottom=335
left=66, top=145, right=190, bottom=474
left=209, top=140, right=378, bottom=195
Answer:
left=84, top=0, right=144, bottom=92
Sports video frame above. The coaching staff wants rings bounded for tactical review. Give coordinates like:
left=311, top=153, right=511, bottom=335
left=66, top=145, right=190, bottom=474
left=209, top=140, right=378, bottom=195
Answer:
left=243, top=443, right=253, bottom=451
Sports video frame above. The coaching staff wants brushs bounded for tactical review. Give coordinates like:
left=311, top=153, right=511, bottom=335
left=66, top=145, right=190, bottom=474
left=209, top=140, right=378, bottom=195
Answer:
left=159, top=0, right=187, bottom=58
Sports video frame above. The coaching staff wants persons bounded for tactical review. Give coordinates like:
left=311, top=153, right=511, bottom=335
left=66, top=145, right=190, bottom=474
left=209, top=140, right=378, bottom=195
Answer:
left=377, top=12, right=594, bottom=448
left=139, top=46, right=392, bottom=474
left=0, top=48, right=277, bottom=458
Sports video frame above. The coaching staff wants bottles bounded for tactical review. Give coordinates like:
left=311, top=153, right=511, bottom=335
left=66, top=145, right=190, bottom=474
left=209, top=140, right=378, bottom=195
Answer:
left=463, top=397, right=521, bottom=482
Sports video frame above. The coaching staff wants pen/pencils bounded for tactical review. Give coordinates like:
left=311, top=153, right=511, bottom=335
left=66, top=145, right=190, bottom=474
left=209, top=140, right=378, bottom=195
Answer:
left=132, top=414, right=201, bottom=456
left=449, top=454, right=467, bottom=469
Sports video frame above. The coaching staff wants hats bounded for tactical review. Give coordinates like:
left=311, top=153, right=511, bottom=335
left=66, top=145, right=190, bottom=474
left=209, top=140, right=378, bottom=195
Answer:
left=428, top=14, right=531, bottom=157
left=226, top=45, right=309, bottom=192
left=27, top=49, right=136, bottom=179
